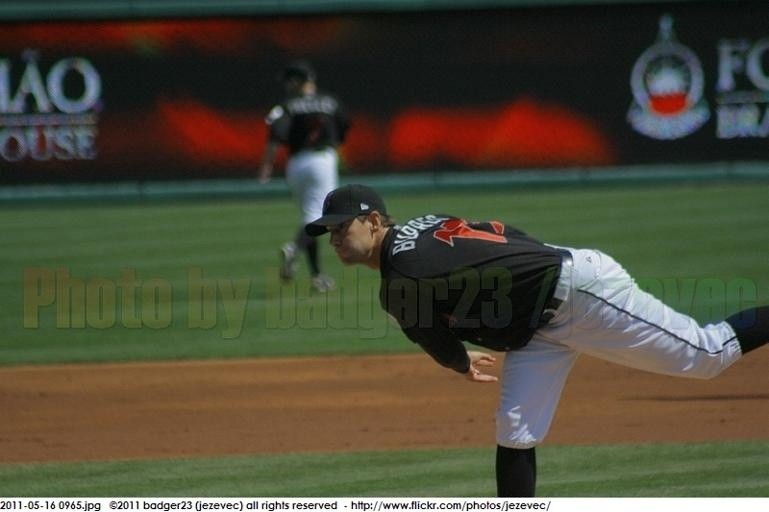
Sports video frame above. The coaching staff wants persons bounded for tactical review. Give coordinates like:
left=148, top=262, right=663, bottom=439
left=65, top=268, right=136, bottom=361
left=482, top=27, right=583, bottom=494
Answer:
left=257, top=63, right=354, bottom=294
left=303, top=183, right=769, bottom=496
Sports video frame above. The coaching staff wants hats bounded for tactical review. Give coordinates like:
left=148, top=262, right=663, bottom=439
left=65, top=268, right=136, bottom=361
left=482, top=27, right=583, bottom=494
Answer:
left=304, top=184, right=387, bottom=237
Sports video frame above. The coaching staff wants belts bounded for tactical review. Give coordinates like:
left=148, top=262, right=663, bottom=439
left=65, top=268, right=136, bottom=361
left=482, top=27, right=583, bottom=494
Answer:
left=536, top=250, right=573, bottom=329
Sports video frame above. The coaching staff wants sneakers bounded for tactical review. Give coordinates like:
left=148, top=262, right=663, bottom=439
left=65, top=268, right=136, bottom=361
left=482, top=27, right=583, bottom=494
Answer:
left=310, top=274, right=335, bottom=294
left=280, top=241, right=297, bottom=282
